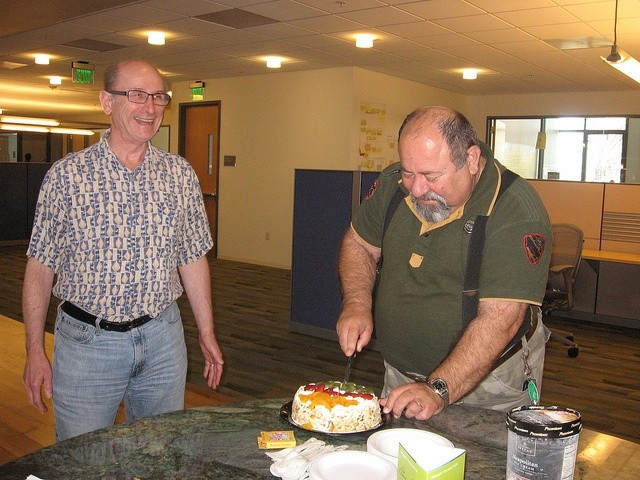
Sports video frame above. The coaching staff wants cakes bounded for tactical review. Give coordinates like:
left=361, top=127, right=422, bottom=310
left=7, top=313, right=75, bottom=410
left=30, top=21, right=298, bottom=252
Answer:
left=291, top=380, right=384, bottom=433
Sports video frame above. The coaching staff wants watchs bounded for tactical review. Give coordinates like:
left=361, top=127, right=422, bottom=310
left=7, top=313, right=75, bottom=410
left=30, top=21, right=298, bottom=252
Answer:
left=421, top=371, right=455, bottom=412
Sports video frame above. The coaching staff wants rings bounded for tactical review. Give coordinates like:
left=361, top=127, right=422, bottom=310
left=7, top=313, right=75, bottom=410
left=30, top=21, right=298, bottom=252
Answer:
left=209, top=360, right=216, bottom=371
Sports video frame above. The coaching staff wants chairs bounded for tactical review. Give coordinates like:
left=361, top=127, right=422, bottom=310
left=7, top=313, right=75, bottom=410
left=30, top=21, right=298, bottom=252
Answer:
left=541, top=225, right=584, bottom=357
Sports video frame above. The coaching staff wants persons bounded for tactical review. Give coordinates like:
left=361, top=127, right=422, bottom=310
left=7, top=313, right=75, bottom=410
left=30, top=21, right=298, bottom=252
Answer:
left=23, top=57, right=224, bottom=443
left=336, top=106, right=554, bottom=409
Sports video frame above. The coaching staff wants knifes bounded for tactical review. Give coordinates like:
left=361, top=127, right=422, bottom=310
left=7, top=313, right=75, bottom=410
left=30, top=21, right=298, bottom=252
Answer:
left=344, top=349, right=358, bottom=381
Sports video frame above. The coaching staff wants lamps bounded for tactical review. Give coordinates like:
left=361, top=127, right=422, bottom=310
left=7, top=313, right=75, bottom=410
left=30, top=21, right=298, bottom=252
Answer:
left=600, top=1, right=640, bottom=83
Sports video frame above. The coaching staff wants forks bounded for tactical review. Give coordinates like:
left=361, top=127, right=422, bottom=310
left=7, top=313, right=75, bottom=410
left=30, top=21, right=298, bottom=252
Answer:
left=266, top=438, right=349, bottom=480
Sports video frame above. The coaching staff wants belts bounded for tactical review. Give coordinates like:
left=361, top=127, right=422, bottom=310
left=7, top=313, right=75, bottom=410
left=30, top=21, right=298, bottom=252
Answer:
left=58, top=302, right=151, bottom=333
left=391, top=306, right=538, bottom=382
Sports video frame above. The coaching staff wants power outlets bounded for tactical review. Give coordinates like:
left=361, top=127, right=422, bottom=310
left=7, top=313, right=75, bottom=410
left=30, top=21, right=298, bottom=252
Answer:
left=224, top=155, right=236, bottom=167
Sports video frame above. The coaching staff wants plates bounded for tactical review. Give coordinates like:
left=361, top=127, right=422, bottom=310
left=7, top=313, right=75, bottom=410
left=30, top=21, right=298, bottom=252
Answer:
left=368, top=429, right=455, bottom=469
left=313, top=451, right=396, bottom=480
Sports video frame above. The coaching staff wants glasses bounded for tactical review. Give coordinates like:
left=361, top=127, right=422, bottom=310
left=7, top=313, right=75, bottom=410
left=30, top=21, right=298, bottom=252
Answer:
left=111, top=90, right=171, bottom=106
